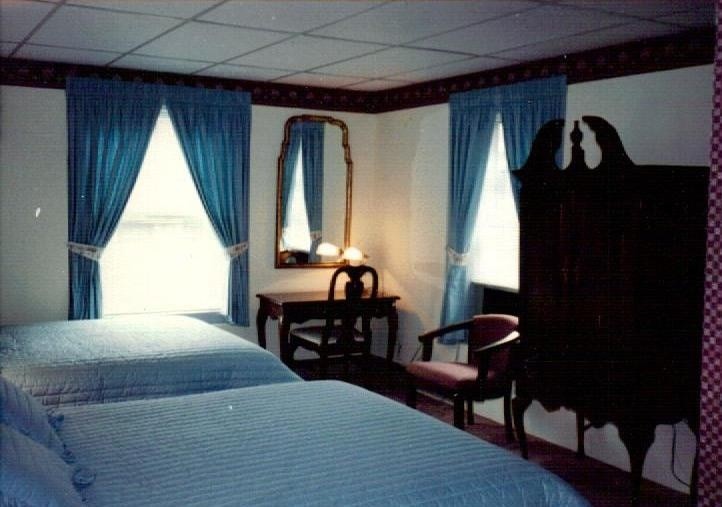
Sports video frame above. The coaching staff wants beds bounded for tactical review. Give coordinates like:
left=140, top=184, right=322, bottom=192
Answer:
left=3, top=313, right=271, bottom=408
left=47, top=380, right=589, bottom=506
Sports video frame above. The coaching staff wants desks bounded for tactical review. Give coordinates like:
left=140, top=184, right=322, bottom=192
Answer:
left=254, top=292, right=401, bottom=366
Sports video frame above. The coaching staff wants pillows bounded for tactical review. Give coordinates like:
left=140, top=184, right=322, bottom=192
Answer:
left=3, top=423, right=96, bottom=507
left=1, top=375, right=77, bottom=459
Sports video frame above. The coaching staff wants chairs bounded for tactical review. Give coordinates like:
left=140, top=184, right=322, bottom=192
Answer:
left=402, top=314, right=519, bottom=446
left=288, top=263, right=380, bottom=381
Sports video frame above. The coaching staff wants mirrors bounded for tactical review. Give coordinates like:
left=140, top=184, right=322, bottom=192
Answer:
left=272, top=113, right=353, bottom=269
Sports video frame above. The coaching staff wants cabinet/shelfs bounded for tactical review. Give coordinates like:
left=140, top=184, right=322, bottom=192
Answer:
left=510, top=111, right=710, bottom=499
